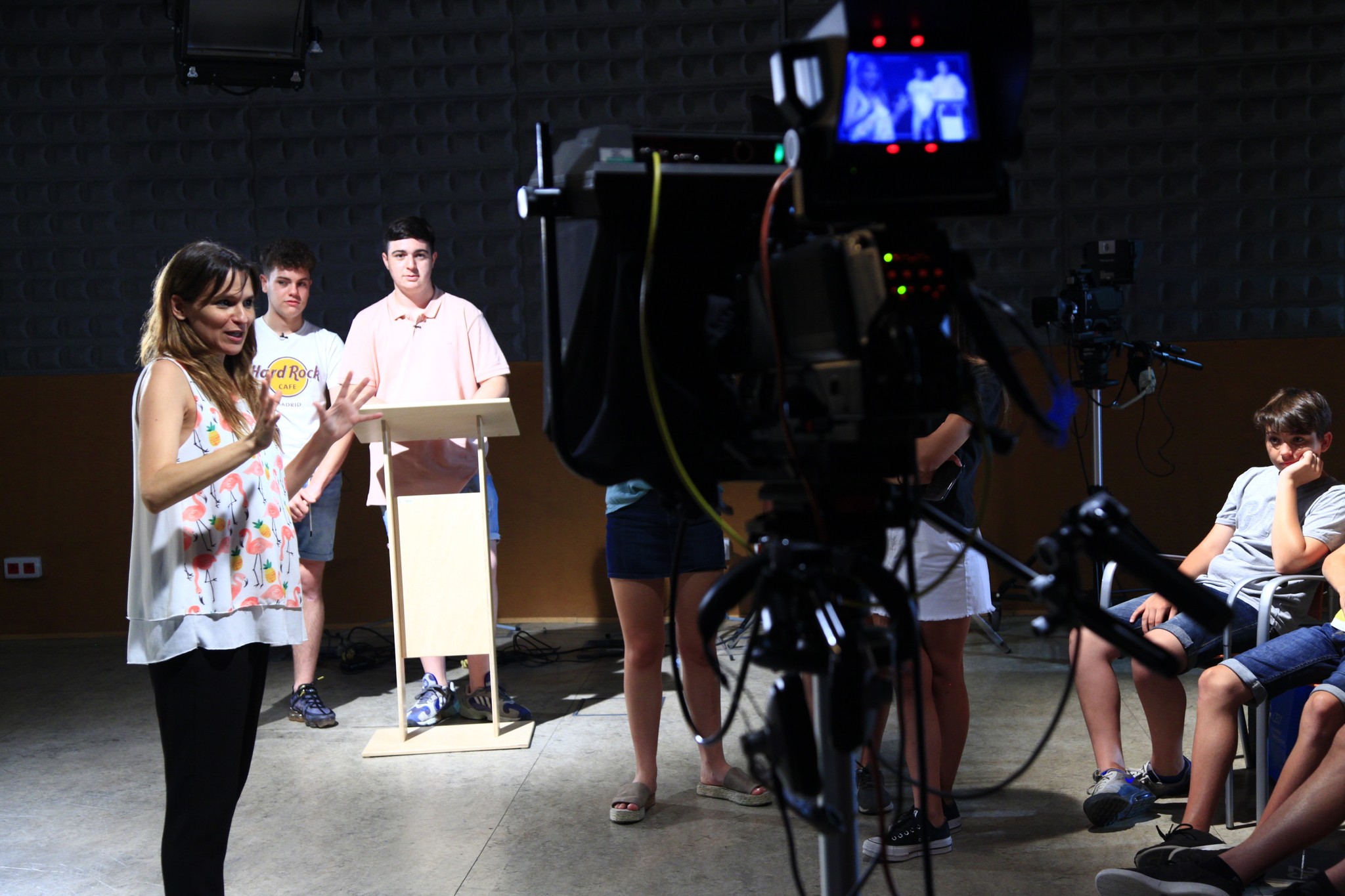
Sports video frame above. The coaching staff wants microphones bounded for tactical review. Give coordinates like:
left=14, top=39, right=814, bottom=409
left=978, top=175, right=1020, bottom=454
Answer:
left=280, top=333, right=288, bottom=338
left=414, top=325, right=421, bottom=328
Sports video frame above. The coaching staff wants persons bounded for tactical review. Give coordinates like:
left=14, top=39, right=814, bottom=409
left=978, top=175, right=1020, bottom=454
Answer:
left=128, top=241, right=388, bottom=896
left=1094, top=541, right=1345, bottom=895
left=862, top=289, right=997, bottom=863
left=844, top=60, right=970, bottom=143
left=603, top=475, right=783, bottom=819
left=252, top=241, right=355, bottom=726
left=1072, top=387, right=1335, bottom=829
left=344, top=219, right=535, bottom=728
left=792, top=587, right=895, bottom=813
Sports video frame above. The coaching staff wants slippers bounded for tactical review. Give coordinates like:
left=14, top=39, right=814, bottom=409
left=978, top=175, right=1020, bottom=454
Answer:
left=697, top=767, right=773, bottom=807
left=610, top=782, right=656, bottom=823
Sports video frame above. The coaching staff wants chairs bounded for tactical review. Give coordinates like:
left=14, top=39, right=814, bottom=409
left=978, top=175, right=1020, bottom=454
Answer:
left=1101, top=549, right=1338, bottom=830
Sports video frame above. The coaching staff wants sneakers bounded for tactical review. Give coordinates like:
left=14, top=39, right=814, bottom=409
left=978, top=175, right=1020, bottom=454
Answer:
left=289, top=675, right=336, bottom=729
left=1083, top=768, right=1156, bottom=829
left=1134, top=824, right=1235, bottom=871
left=407, top=672, right=455, bottom=727
left=1125, top=756, right=1192, bottom=799
left=888, top=799, right=962, bottom=834
left=1095, top=848, right=1246, bottom=896
left=863, top=806, right=953, bottom=862
left=855, top=759, right=893, bottom=814
left=461, top=672, right=532, bottom=722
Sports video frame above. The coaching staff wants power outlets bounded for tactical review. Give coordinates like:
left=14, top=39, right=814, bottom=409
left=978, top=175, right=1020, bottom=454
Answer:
left=4, top=557, right=43, bottom=579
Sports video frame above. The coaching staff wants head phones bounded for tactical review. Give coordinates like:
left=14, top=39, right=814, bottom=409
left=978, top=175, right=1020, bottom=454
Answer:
left=695, top=545, right=921, bottom=795
